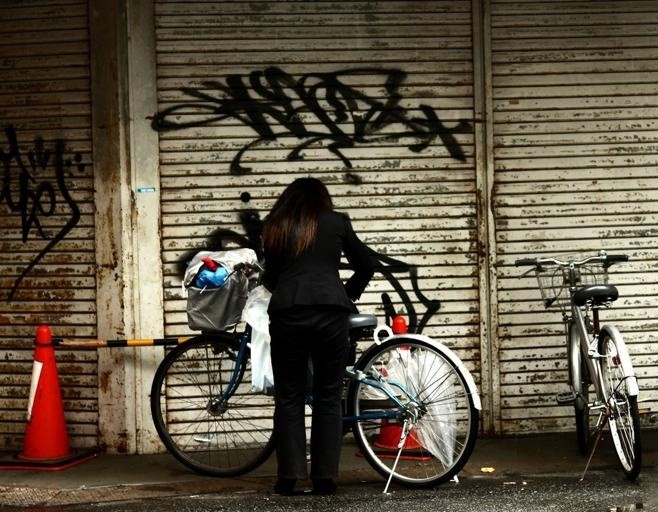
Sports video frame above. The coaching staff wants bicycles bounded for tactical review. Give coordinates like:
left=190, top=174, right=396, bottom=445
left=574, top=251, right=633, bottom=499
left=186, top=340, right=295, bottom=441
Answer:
left=513, top=249, right=643, bottom=489
left=148, top=259, right=484, bottom=497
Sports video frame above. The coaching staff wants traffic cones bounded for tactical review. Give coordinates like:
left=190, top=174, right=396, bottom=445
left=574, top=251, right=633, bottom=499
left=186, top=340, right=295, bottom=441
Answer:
left=0, top=324, right=100, bottom=475
left=351, top=315, right=434, bottom=465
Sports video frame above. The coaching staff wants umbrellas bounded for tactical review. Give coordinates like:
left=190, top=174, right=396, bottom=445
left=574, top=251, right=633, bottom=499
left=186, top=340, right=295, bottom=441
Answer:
left=372, top=322, right=461, bottom=486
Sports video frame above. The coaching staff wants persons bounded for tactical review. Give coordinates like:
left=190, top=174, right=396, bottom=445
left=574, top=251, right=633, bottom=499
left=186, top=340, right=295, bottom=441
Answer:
left=256, top=176, right=375, bottom=497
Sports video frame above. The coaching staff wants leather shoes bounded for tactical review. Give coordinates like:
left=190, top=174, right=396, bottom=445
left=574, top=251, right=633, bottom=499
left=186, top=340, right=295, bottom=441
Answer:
left=273, top=479, right=339, bottom=495
